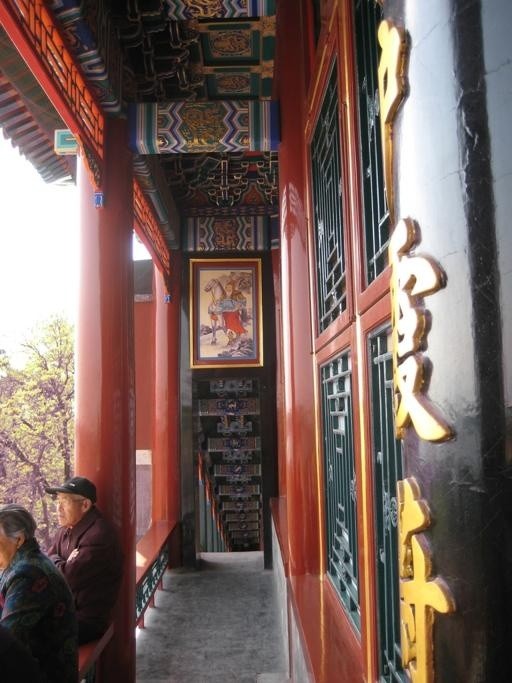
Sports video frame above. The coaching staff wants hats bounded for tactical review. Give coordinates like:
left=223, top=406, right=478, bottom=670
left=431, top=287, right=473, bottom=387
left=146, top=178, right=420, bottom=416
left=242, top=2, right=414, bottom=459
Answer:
left=45, top=477, right=96, bottom=503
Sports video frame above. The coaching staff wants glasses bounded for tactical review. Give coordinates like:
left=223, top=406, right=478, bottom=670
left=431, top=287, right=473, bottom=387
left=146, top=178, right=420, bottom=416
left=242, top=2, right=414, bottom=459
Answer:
left=52, top=498, right=85, bottom=506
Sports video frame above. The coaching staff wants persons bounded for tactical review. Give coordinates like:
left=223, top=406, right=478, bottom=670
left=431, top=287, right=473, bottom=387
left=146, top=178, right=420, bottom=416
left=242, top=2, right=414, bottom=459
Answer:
left=223, top=282, right=247, bottom=338
left=0, top=503, right=79, bottom=683
left=45, top=476, right=121, bottom=645
